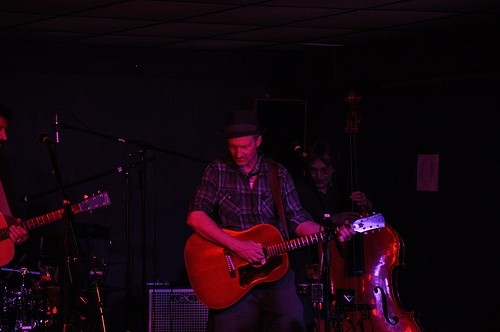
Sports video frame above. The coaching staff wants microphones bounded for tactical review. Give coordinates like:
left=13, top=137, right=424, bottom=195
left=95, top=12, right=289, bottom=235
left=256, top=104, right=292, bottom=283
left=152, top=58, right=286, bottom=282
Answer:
left=54, top=111, right=60, bottom=144
left=289, top=141, right=316, bottom=164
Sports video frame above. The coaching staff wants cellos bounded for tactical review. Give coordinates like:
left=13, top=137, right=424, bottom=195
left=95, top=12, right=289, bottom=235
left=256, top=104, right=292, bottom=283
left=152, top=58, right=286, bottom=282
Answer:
left=312, top=90, right=426, bottom=332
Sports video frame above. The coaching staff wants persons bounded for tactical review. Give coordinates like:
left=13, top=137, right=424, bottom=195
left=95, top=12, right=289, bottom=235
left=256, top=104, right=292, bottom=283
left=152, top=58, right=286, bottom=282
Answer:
left=186, top=110, right=357, bottom=332
left=288, top=140, right=372, bottom=283
left=0, top=116, right=29, bottom=268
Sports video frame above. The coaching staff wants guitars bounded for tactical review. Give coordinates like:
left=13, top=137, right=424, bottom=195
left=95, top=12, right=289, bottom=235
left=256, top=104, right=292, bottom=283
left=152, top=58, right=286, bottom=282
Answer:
left=184, top=212, right=386, bottom=309
left=0, top=191, right=111, bottom=267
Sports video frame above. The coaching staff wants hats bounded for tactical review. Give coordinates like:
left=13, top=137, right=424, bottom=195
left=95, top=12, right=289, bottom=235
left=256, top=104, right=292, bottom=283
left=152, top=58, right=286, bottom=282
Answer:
left=225, top=110, right=260, bottom=138
